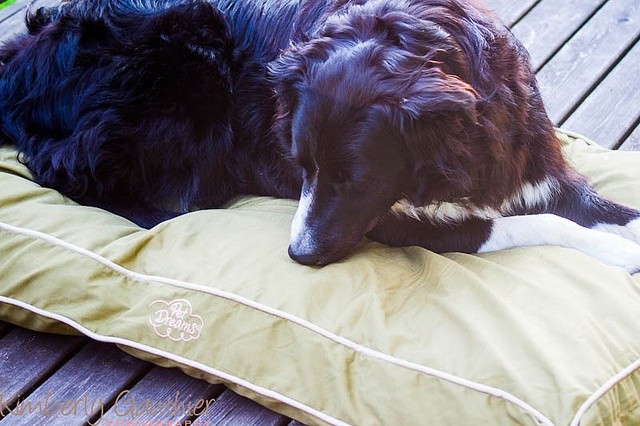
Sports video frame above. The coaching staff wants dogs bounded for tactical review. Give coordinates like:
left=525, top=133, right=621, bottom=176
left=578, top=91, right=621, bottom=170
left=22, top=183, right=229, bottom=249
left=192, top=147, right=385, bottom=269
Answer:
left=0, top=1, right=640, bottom=279
